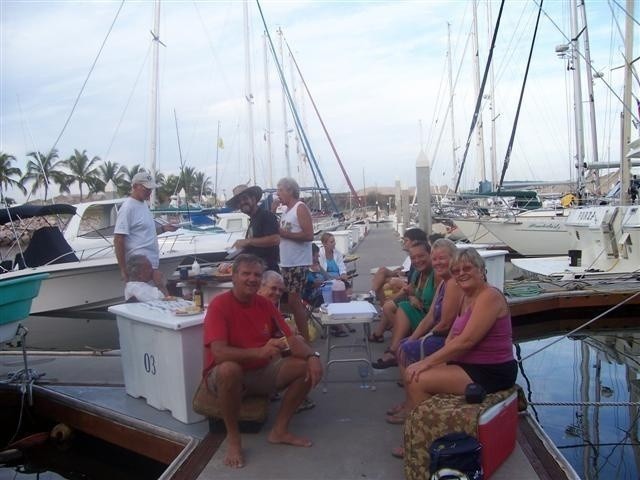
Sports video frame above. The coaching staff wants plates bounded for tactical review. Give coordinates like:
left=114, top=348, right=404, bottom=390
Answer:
left=175, top=312, right=201, bottom=316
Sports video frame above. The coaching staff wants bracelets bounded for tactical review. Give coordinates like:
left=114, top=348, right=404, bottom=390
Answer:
left=161, top=226, right=165, bottom=232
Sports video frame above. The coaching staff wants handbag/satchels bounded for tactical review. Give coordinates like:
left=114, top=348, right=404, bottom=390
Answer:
left=429, top=431, right=484, bottom=480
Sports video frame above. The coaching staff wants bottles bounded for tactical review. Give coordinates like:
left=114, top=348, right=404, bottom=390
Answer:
left=193, top=277, right=205, bottom=311
left=192, top=256, right=201, bottom=274
left=333, top=278, right=346, bottom=302
left=270, top=317, right=292, bottom=359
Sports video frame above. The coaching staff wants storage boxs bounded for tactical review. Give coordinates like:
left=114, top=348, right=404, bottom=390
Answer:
left=403, top=382, right=521, bottom=480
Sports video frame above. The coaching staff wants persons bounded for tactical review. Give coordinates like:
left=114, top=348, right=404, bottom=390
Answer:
left=362, top=228, right=519, bottom=459
left=199, top=253, right=324, bottom=468
left=218, top=184, right=280, bottom=274
left=123, top=254, right=171, bottom=302
left=318, top=232, right=352, bottom=285
left=301, top=242, right=354, bottom=337
left=255, top=269, right=318, bottom=415
left=112, top=172, right=181, bottom=283
left=270, top=177, right=314, bottom=346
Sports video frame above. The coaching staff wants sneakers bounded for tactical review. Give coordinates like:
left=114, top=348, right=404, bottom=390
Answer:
left=385, top=403, right=406, bottom=423
left=392, top=446, right=404, bottom=457
left=294, top=397, right=316, bottom=413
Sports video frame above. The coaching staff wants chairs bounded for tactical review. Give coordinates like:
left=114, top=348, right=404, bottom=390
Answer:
left=126, top=296, right=138, bottom=302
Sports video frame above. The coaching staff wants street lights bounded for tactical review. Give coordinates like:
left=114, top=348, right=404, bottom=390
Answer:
left=376, top=200, right=379, bottom=221
left=387, top=202, right=389, bottom=216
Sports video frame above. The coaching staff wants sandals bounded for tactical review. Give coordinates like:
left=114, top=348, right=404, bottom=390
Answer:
left=363, top=332, right=385, bottom=343
left=373, top=347, right=398, bottom=369
left=331, top=325, right=356, bottom=337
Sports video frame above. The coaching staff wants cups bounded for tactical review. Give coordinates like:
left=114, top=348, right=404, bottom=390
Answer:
left=384, top=290, right=392, bottom=298
left=180, top=267, right=188, bottom=280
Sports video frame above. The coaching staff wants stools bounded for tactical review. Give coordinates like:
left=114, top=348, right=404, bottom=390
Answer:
left=295, top=295, right=325, bottom=341
left=191, top=376, right=270, bottom=434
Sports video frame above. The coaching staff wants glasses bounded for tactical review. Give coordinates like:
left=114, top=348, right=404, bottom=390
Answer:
left=452, top=265, right=474, bottom=275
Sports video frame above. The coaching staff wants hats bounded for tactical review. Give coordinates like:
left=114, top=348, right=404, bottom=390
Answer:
left=132, top=172, right=162, bottom=190
left=225, top=184, right=263, bottom=210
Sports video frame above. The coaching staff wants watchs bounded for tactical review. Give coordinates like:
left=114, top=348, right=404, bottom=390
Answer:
left=305, top=351, right=321, bottom=361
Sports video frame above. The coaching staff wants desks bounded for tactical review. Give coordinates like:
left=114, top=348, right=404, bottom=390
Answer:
left=106, top=300, right=209, bottom=425
left=175, top=281, right=234, bottom=304
left=317, top=302, right=382, bottom=390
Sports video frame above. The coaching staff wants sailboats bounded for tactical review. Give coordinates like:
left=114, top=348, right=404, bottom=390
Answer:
left=514, top=329, right=639, bottom=479
